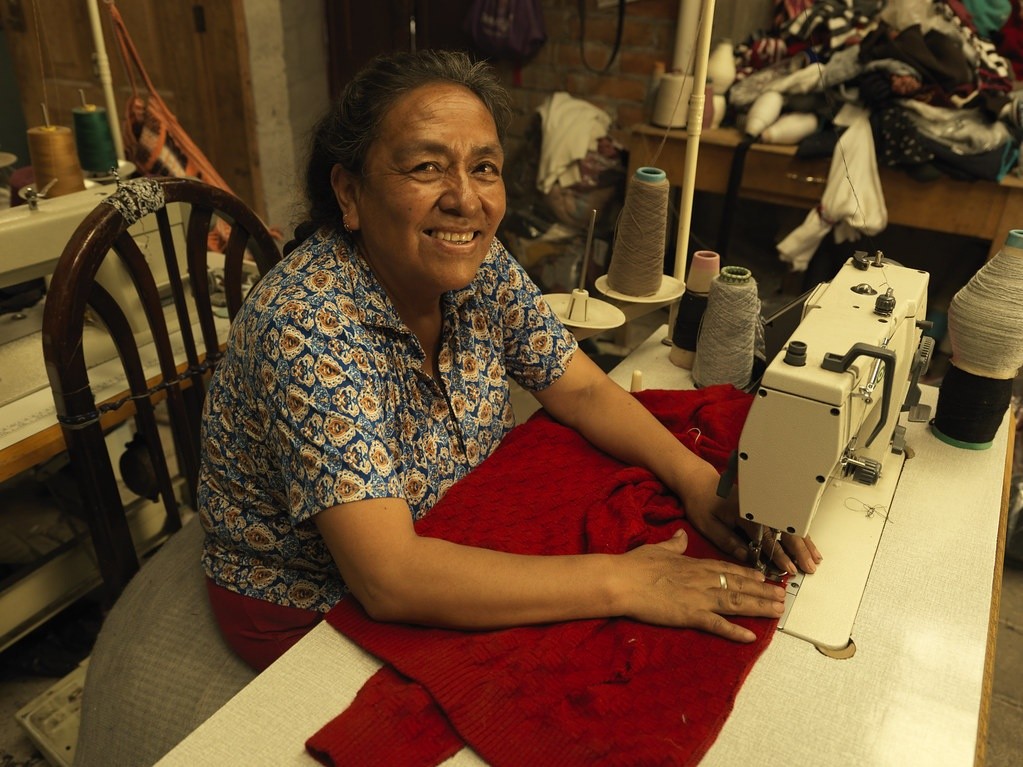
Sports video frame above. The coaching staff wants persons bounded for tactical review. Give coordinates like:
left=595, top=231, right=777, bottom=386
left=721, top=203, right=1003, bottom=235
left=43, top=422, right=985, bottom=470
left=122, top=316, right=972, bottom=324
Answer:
left=197, top=46, right=826, bottom=678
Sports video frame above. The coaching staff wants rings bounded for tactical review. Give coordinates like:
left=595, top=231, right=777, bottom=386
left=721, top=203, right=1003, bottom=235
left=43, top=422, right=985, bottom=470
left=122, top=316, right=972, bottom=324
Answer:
left=718, top=572, right=731, bottom=591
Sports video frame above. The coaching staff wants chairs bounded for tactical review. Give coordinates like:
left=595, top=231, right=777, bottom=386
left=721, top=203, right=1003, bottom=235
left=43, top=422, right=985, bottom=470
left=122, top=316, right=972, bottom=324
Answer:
left=42, top=176, right=284, bottom=606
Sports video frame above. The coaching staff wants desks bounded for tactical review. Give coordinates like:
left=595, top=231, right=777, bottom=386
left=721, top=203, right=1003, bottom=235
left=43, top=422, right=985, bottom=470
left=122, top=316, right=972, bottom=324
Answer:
left=623, top=122, right=1022, bottom=269
left=153, top=323, right=1017, bottom=767
